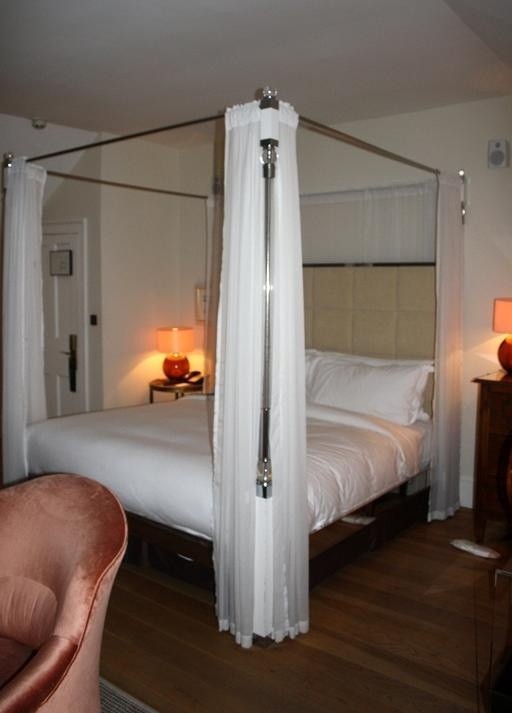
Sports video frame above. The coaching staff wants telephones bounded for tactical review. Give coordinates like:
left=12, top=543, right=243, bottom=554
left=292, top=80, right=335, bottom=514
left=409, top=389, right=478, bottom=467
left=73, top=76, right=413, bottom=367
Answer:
left=183, top=371, right=203, bottom=384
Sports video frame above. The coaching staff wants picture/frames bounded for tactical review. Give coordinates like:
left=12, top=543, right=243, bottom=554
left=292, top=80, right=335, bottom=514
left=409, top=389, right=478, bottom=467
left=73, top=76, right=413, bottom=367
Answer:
left=194, top=286, right=205, bottom=324
left=47, top=249, right=73, bottom=276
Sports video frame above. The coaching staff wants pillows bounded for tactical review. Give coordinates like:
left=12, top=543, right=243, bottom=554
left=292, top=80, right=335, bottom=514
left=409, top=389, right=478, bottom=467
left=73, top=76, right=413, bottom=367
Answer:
left=303, top=349, right=436, bottom=425
left=0, top=574, right=58, bottom=651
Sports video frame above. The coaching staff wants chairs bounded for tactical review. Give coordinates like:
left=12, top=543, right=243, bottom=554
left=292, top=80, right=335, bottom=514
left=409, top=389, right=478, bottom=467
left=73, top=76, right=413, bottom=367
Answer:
left=0, top=469, right=131, bottom=712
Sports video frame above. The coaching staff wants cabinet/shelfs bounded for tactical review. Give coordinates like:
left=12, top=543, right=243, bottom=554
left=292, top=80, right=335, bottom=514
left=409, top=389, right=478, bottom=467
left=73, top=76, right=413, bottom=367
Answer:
left=469, top=371, right=512, bottom=551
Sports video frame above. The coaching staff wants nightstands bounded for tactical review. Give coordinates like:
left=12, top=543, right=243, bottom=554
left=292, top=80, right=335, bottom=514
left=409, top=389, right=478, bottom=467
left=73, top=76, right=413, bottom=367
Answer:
left=148, top=380, right=203, bottom=403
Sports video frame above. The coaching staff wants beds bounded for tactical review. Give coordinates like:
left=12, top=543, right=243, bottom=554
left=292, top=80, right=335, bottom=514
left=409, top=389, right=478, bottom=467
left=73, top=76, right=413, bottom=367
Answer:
left=22, top=346, right=445, bottom=647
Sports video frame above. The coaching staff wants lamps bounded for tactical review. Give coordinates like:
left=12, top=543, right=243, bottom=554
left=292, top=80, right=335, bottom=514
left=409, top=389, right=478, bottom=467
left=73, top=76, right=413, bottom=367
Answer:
left=492, top=297, right=511, bottom=376
left=155, top=327, right=195, bottom=380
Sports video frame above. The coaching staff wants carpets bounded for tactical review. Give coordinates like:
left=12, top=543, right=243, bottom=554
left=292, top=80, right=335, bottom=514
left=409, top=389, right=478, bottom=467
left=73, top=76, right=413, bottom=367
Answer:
left=99, top=675, right=163, bottom=712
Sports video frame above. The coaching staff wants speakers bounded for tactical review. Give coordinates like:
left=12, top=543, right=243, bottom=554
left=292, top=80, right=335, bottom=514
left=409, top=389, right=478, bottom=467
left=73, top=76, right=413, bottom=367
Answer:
left=488, top=141, right=507, bottom=168
